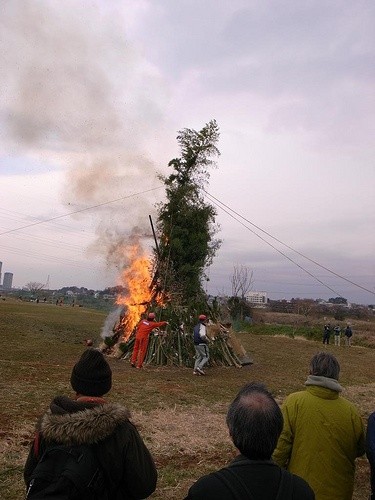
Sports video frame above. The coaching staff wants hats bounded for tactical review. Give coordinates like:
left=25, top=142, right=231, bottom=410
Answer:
left=70, top=350, right=112, bottom=397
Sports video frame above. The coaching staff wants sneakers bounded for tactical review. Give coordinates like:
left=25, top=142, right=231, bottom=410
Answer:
left=193, top=367, right=205, bottom=376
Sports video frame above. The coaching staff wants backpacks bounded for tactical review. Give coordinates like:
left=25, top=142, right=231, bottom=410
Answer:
left=26, top=430, right=110, bottom=500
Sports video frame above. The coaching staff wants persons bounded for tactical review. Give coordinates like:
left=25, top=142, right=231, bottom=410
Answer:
left=183, top=381, right=315, bottom=500
left=56, top=299, right=59, bottom=305
left=345, top=325, right=353, bottom=348
left=44, top=298, right=47, bottom=303
left=192, top=316, right=212, bottom=375
left=37, top=299, right=39, bottom=303
left=365, top=409, right=375, bottom=500
left=322, top=323, right=342, bottom=346
left=271, top=353, right=366, bottom=500
left=131, top=313, right=169, bottom=370
left=23, top=348, right=159, bottom=500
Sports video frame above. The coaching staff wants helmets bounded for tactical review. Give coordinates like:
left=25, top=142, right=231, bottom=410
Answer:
left=148, top=313, right=156, bottom=318
left=199, top=315, right=206, bottom=320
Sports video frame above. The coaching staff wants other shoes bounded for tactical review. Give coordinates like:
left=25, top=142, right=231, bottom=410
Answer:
left=131, top=363, right=135, bottom=367
left=136, top=366, right=140, bottom=369
